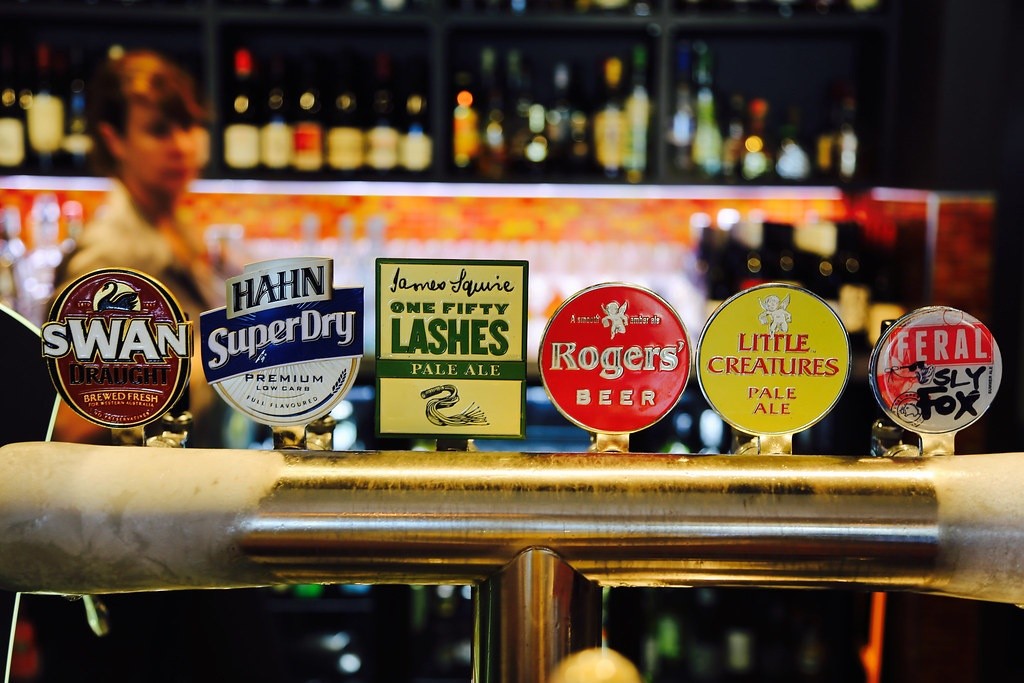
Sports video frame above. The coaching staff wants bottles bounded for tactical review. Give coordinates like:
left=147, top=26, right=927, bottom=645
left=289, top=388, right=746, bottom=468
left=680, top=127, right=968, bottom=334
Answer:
left=440, top=42, right=654, bottom=186
left=219, top=45, right=435, bottom=181
left=0, top=39, right=103, bottom=177
left=664, top=36, right=866, bottom=187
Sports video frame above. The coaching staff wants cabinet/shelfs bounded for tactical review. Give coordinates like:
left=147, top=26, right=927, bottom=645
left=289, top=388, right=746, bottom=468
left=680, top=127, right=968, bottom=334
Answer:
left=0, top=0, right=909, bottom=190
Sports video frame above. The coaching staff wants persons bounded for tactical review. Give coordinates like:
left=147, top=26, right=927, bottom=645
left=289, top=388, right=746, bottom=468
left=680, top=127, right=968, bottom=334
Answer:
left=35, top=55, right=242, bottom=683
